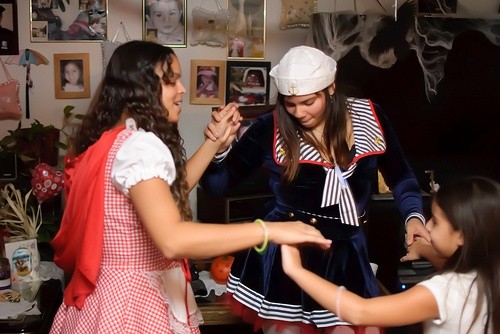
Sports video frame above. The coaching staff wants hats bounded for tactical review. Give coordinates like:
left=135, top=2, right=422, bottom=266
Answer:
left=269, top=45, right=337, bottom=98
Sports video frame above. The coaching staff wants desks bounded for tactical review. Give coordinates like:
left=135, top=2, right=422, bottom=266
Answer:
left=199, top=279, right=244, bottom=325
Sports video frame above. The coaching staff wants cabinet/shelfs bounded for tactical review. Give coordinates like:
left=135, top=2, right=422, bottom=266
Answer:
left=196, top=186, right=275, bottom=224
left=362, top=189, right=434, bottom=294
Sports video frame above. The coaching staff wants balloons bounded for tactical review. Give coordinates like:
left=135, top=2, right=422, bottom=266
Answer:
left=32, top=162, right=65, bottom=204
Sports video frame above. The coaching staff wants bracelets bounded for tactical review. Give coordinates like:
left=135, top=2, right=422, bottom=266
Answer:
left=254, top=218, right=268, bottom=254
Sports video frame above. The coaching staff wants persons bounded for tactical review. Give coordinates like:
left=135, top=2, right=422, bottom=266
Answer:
left=197, top=67, right=218, bottom=98
left=62, top=61, right=84, bottom=92
left=0, top=5, right=13, bottom=51
left=281, top=171, right=500, bottom=333
left=49, top=40, right=334, bottom=334
left=204, top=45, right=435, bottom=334
left=145, top=0, right=185, bottom=43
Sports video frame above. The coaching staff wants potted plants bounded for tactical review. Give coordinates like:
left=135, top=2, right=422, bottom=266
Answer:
left=0, top=104, right=86, bottom=175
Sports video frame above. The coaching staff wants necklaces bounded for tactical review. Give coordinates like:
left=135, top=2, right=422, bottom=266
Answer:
left=314, top=127, right=324, bottom=144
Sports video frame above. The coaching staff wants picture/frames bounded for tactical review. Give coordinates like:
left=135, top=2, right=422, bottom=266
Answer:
left=226, top=61, right=271, bottom=107
left=0, top=0, right=19, bottom=56
left=279, top=0, right=318, bottom=30
left=29, top=0, right=109, bottom=43
left=189, top=59, right=226, bottom=105
left=226, top=0, right=266, bottom=61
left=142, top=0, right=188, bottom=48
left=53, top=53, right=92, bottom=99
